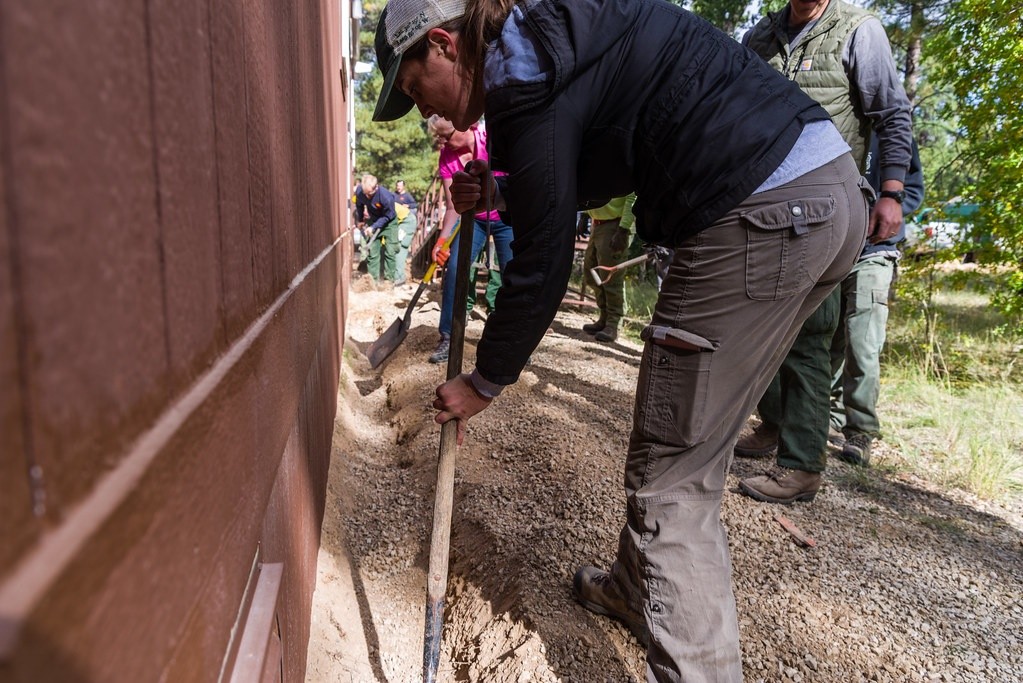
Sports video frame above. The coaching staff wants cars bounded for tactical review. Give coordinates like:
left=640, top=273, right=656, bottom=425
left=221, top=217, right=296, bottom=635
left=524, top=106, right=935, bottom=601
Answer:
left=918, top=196, right=985, bottom=251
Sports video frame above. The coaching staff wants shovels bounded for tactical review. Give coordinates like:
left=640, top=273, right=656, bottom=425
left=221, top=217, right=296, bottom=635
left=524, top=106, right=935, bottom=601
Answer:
left=361, top=225, right=381, bottom=249
left=365, top=214, right=462, bottom=371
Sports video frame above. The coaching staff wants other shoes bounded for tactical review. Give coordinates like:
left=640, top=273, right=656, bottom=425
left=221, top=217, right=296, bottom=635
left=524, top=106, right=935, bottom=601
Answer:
left=827, top=425, right=847, bottom=447
left=573, top=566, right=652, bottom=650
left=841, top=433, right=871, bottom=464
left=733, top=424, right=780, bottom=457
left=583, top=320, right=606, bottom=335
left=738, top=463, right=821, bottom=504
left=595, top=327, right=617, bottom=342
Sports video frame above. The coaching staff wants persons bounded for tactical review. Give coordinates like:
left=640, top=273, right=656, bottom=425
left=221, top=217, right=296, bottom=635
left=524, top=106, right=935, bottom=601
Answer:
left=372, top=0, right=877, bottom=683
left=352, top=114, right=639, bottom=366
left=733, top=0, right=926, bottom=505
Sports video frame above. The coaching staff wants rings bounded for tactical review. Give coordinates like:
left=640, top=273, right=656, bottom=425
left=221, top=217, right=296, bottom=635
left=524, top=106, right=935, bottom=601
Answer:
left=890, top=231, right=898, bottom=236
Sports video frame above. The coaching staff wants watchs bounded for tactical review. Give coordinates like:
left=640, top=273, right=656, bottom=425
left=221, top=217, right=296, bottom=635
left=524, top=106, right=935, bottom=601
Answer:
left=879, top=189, right=912, bottom=205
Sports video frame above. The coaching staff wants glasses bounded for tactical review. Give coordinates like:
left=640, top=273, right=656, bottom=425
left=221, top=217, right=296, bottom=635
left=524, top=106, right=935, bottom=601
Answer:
left=432, top=129, right=457, bottom=141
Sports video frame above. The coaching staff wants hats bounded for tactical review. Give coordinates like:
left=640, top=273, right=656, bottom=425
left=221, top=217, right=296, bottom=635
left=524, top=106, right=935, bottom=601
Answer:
left=373, top=0, right=469, bottom=122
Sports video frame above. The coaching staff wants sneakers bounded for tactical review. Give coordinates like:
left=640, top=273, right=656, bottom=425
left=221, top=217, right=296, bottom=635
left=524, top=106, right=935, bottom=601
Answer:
left=428, top=334, right=450, bottom=363
